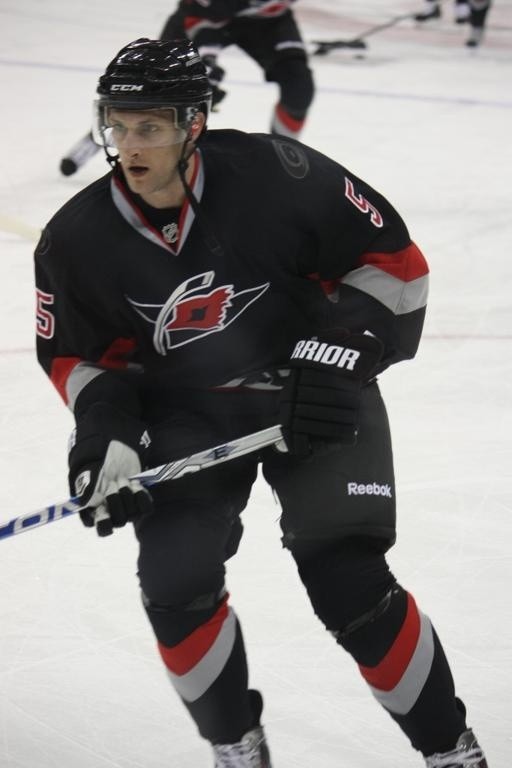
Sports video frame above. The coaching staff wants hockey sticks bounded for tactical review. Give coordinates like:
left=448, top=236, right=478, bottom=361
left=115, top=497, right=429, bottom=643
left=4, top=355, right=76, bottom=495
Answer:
left=309, top=1, right=436, bottom=63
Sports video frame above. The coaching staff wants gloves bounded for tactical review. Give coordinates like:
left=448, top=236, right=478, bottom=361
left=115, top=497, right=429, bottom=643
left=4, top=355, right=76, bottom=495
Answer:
left=68, top=400, right=157, bottom=537
left=275, top=328, right=384, bottom=461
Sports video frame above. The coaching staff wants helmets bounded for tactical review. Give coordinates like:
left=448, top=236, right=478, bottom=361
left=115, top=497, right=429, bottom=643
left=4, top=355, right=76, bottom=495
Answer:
left=94, top=38, right=212, bottom=148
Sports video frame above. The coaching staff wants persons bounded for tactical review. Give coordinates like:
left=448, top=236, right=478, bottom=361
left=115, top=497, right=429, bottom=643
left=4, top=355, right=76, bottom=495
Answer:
left=32, top=36, right=489, bottom=768
left=61, top=0, right=314, bottom=179
left=416, top=0, right=491, bottom=46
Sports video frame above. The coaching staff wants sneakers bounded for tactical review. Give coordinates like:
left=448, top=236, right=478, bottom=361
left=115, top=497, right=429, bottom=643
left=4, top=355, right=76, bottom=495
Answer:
left=212, top=722, right=270, bottom=767
left=423, top=726, right=488, bottom=767
